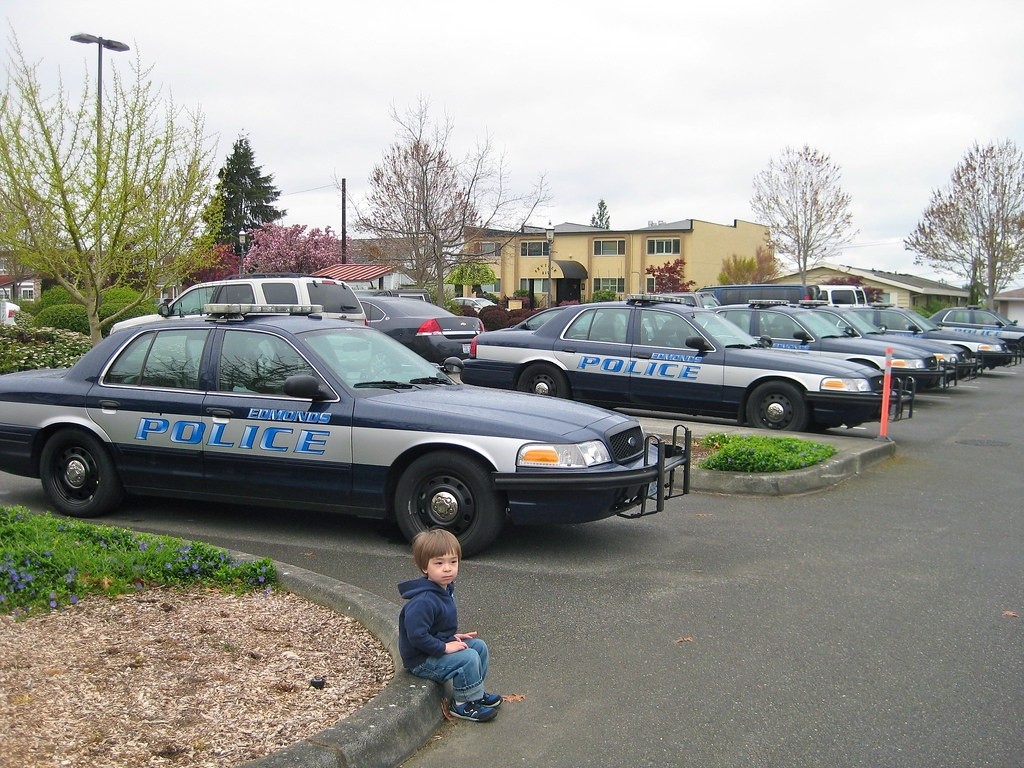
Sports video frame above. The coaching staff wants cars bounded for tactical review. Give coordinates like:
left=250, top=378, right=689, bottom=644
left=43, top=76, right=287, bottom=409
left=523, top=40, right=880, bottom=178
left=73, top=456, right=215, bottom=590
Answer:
left=654, top=282, right=1024, bottom=389
left=461, top=293, right=916, bottom=432
left=446, top=298, right=511, bottom=314
left=497, top=305, right=649, bottom=346
left=356, top=296, right=485, bottom=366
left=0, top=298, right=20, bottom=325
left=0, top=302, right=694, bottom=560
left=374, top=289, right=431, bottom=304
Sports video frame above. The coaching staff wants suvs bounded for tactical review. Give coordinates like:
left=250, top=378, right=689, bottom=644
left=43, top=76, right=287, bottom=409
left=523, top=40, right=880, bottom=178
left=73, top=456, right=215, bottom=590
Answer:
left=110, top=272, right=371, bottom=373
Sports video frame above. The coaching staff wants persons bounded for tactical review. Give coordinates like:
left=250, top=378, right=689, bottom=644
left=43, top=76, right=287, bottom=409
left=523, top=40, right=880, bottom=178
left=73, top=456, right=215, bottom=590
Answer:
left=396, top=530, right=504, bottom=722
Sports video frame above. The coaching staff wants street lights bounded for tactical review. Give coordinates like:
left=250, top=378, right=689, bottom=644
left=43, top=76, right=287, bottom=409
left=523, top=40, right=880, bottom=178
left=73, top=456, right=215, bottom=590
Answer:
left=239, top=228, right=247, bottom=274
left=545, top=219, right=555, bottom=308
left=70, top=33, right=130, bottom=285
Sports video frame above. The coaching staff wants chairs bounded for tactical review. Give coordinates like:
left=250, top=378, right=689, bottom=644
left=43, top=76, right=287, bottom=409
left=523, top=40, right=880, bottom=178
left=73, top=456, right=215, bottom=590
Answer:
left=271, top=349, right=299, bottom=382
left=770, top=315, right=784, bottom=339
left=661, top=320, right=679, bottom=348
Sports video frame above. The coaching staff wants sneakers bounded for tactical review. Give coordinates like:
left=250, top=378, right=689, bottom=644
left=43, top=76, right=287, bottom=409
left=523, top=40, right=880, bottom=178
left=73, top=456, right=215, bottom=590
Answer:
left=477, top=691, right=503, bottom=707
left=449, top=698, right=498, bottom=721
